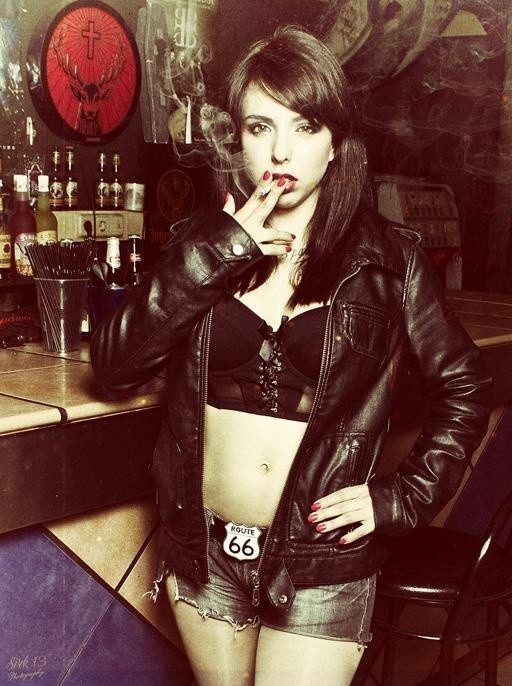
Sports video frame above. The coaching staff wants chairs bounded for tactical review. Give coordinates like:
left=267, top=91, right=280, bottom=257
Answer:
left=357, top=488, right=512, bottom=683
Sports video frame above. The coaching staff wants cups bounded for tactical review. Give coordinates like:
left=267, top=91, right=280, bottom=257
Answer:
left=125, top=182, right=146, bottom=209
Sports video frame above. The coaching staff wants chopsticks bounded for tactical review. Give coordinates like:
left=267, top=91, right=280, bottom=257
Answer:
left=23, top=238, right=98, bottom=352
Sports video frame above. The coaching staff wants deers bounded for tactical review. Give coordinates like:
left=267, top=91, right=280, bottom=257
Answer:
left=53, top=29, right=129, bottom=138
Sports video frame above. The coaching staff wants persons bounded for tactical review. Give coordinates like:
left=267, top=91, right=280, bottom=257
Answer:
left=89, top=23, right=495, bottom=686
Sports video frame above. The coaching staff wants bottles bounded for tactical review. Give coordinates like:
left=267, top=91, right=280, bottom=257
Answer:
left=51, top=141, right=80, bottom=209
left=94, top=149, right=124, bottom=209
left=104, top=234, right=148, bottom=305
left=0, top=175, right=61, bottom=278
